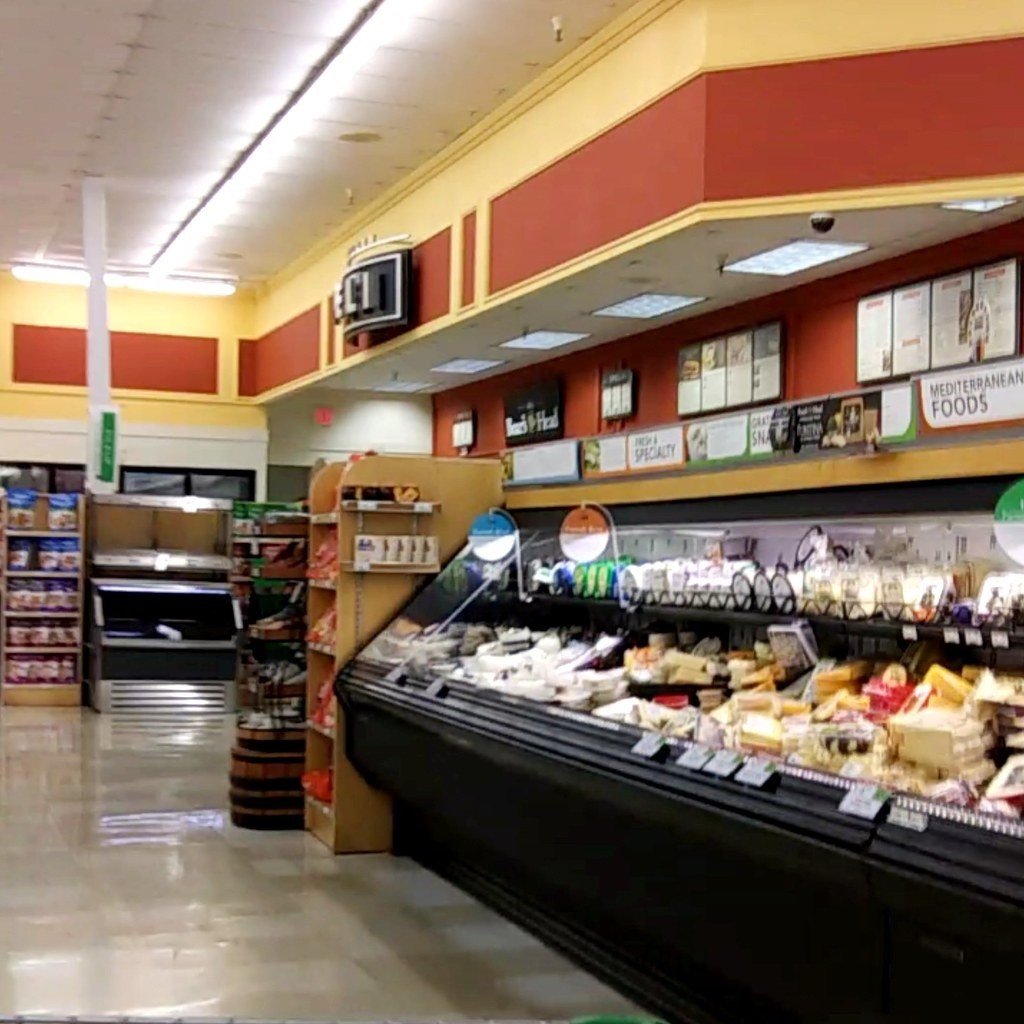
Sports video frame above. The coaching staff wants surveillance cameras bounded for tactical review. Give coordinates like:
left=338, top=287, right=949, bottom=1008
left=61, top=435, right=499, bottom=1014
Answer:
left=809, top=212, right=835, bottom=234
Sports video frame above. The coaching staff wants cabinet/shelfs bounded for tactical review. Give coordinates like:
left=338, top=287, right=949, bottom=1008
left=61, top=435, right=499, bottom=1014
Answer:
left=227, top=523, right=309, bottom=832
left=302, top=455, right=506, bottom=856
left=332, top=531, right=1024, bottom=1024
left=0, top=487, right=87, bottom=706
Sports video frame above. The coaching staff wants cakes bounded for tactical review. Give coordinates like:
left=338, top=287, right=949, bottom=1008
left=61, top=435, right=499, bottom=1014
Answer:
left=467, top=629, right=1024, bottom=817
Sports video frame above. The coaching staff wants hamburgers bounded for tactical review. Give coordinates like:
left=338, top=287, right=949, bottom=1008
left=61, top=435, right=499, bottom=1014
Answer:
left=682, top=361, right=698, bottom=375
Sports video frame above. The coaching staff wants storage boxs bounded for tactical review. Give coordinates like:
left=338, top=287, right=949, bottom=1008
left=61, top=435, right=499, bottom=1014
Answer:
left=353, top=533, right=386, bottom=563
left=397, top=535, right=412, bottom=562
left=424, top=536, right=440, bottom=563
left=384, top=535, right=400, bottom=562
left=411, top=535, right=426, bottom=563
